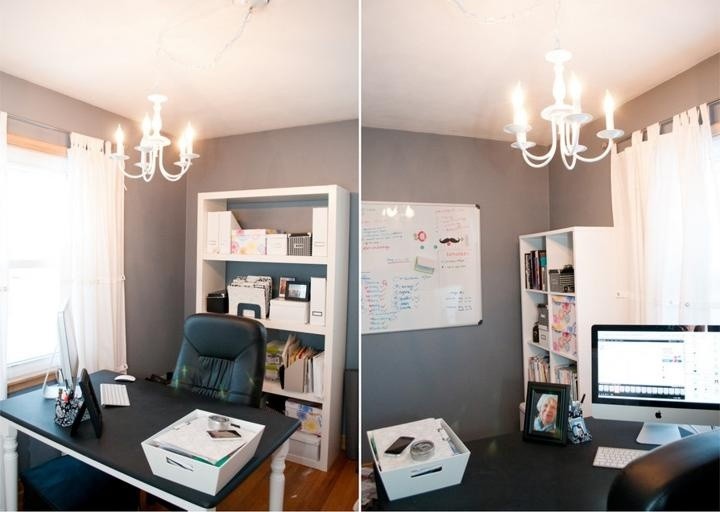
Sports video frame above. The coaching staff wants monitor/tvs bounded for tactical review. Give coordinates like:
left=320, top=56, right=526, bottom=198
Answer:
left=589, top=323, right=720, bottom=445
left=41, top=296, right=82, bottom=399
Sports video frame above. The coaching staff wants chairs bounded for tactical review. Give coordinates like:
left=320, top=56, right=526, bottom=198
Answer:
left=168, top=312, right=267, bottom=408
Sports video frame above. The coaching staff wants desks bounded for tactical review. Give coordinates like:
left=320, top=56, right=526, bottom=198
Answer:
left=0, top=368, right=302, bottom=511
left=373, top=416, right=697, bottom=510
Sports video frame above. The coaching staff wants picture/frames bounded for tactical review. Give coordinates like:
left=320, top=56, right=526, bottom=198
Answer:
left=521, top=380, right=571, bottom=445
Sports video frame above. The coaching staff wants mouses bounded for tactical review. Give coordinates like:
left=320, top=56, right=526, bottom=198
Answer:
left=113, top=374, right=137, bottom=382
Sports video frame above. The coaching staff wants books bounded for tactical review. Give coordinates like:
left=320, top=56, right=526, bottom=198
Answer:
left=264, top=333, right=324, bottom=398
left=523, top=249, right=577, bottom=402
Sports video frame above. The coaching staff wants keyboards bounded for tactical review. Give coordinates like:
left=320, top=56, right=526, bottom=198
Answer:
left=100, top=383, right=131, bottom=407
left=592, top=446, right=650, bottom=469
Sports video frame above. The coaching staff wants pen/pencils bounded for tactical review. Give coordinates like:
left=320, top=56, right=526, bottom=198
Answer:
left=578, top=394, right=586, bottom=409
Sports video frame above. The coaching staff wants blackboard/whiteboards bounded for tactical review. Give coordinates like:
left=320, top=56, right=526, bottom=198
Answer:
left=361, top=201, right=483, bottom=336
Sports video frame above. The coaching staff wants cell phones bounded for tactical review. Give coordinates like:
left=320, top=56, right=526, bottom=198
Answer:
left=384, top=435, right=415, bottom=456
left=206, top=428, right=242, bottom=440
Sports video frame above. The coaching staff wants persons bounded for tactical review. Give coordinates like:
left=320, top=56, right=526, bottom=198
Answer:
left=531, top=394, right=558, bottom=433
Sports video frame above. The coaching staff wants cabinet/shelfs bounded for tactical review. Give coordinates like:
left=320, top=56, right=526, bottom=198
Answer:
left=194, top=184, right=350, bottom=471
left=517, top=223, right=618, bottom=431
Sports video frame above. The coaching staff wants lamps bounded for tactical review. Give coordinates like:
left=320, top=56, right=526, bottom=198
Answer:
left=504, top=47, right=625, bottom=170
left=112, top=94, right=200, bottom=183
